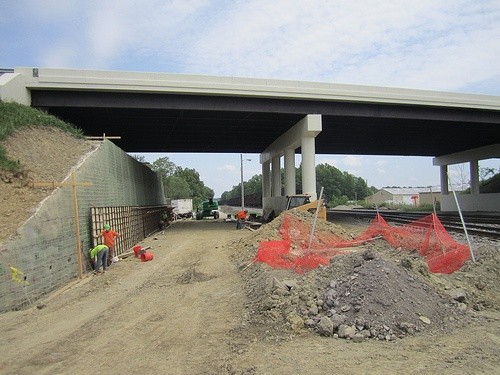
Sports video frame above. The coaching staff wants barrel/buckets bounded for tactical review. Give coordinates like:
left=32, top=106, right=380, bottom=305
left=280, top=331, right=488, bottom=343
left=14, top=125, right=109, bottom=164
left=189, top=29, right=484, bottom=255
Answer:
left=134, top=245, right=142, bottom=257
left=138, top=250, right=146, bottom=258
left=141, top=252, right=153, bottom=261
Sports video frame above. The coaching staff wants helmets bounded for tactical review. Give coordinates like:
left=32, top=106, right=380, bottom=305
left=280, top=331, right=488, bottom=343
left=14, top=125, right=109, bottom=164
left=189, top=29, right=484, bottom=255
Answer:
left=89, top=248, right=92, bottom=253
left=105, top=224, right=110, bottom=231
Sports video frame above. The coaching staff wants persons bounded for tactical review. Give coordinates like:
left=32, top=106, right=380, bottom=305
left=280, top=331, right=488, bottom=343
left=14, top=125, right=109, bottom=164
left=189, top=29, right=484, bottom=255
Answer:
left=236, top=210, right=248, bottom=229
left=92, top=224, right=120, bottom=266
left=89, top=244, right=109, bottom=273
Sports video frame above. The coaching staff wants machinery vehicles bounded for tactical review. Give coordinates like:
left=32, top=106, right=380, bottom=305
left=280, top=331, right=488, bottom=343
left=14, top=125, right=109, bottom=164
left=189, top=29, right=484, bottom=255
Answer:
left=196, top=197, right=219, bottom=220
left=285, top=194, right=326, bottom=221
